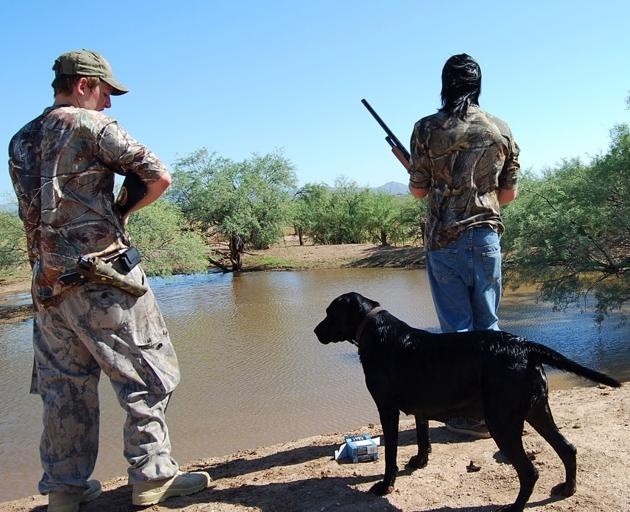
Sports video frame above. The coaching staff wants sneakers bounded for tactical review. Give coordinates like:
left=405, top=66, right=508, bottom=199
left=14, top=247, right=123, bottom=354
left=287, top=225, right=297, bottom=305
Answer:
left=47, top=479, right=102, bottom=511
left=133, top=469, right=210, bottom=506
left=446, top=415, right=490, bottom=437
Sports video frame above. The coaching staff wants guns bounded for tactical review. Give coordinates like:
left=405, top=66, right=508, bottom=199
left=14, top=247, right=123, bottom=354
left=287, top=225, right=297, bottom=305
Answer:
left=361, top=100, right=410, bottom=170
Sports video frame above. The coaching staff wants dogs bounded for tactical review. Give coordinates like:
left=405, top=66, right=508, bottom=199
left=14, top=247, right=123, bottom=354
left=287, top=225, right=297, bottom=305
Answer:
left=313, top=291, right=624, bottom=512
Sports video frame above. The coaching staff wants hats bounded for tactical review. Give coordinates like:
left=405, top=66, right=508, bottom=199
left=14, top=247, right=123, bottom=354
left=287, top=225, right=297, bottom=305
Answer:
left=51, top=48, right=130, bottom=95
left=442, top=53, right=481, bottom=86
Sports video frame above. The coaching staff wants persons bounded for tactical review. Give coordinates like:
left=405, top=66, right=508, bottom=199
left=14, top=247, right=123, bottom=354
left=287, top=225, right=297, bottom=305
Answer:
left=406, top=49, right=519, bottom=442
left=6, top=47, right=214, bottom=511
